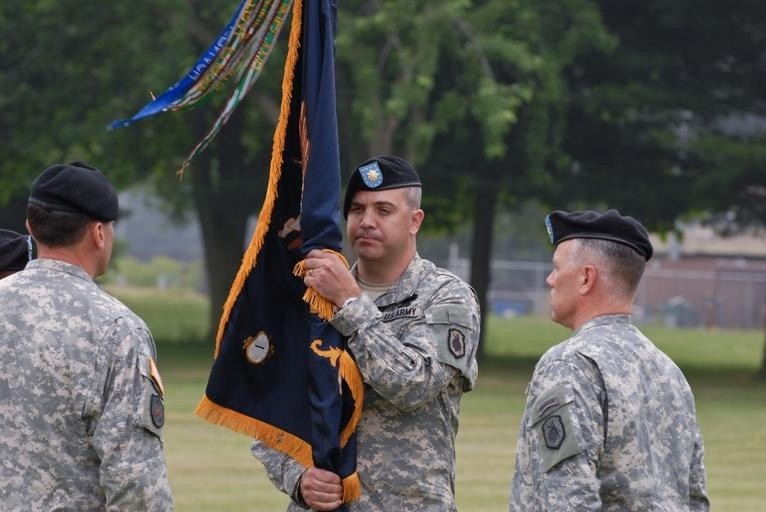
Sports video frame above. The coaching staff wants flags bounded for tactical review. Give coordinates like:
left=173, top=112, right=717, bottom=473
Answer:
left=195, top=0, right=363, bottom=502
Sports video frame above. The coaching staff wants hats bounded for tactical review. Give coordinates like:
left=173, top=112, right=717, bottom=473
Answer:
left=343, top=156, right=422, bottom=220
left=544, top=209, right=653, bottom=261
left=28, top=162, right=118, bottom=221
left=0, top=228, right=32, bottom=272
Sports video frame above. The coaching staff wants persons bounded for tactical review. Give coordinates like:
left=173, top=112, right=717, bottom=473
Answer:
left=510, top=209, right=710, bottom=511
left=1, top=160, right=170, bottom=511
left=252, top=154, right=482, bottom=511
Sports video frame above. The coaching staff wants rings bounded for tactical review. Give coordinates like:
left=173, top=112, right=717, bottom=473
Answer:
left=309, top=269, right=312, bottom=276
